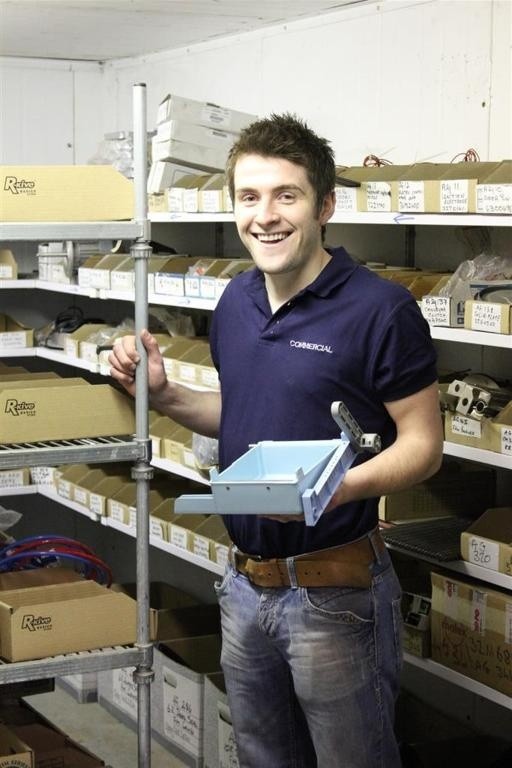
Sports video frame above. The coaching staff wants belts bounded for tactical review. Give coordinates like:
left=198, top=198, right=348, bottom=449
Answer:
left=227, top=529, right=386, bottom=589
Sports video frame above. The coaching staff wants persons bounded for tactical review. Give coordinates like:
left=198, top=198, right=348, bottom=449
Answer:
left=108, top=115, right=446, bottom=765
